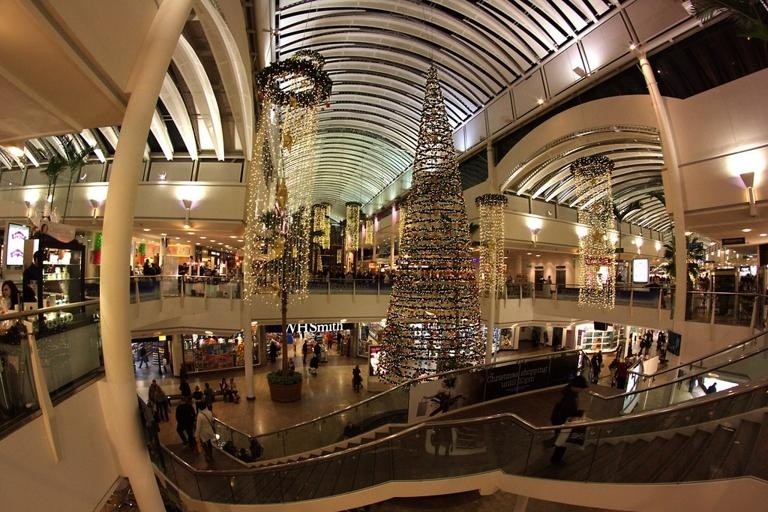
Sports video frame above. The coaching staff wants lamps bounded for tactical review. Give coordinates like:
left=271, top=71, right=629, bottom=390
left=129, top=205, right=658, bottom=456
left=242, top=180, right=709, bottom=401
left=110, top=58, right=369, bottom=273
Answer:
left=740, top=172, right=757, bottom=216
left=90, top=199, right=99, bottom=219
left=25, top=201, right=31, bottom=218
left=573, top=67, right=590, bottom=79
left=182, top=199, right=192, bottom=227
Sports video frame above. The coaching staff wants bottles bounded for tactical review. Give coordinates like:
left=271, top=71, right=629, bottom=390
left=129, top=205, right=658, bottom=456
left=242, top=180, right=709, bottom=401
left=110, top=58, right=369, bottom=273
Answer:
left=43, top=267, right=69, bottom=279
left=1, top=297, right=19, bottom=315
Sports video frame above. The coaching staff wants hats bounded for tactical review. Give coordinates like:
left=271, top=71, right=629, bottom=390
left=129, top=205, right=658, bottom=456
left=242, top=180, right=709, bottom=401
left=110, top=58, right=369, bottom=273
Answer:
left=569, top=376, right=589, bottom=389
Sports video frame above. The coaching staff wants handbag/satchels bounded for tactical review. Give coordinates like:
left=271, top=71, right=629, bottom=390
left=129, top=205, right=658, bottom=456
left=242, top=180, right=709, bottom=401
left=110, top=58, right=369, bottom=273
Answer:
left=555, top=418, right=591, bottom=452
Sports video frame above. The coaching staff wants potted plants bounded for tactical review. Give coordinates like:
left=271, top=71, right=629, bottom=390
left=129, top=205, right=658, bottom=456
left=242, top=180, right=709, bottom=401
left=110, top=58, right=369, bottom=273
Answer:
left=658, top=231, right=704, bottom=305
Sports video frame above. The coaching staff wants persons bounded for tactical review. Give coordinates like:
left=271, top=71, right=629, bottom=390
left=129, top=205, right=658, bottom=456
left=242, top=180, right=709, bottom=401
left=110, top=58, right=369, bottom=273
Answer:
left=148, top=363, right=260, bottom=469
left=23, top=252, right=41, bottom=301
left=591, top=329, right=665, bottom=389
left=650, top=273, right=710, bottom=305
left=538, top=275, right=545, bottom=291
left=353, top=364, right=361, bottom=393
left=616, top=273, right=622, bottom=281
left=543, top=376, right=586, bottom=468
left=688, top=377, right=695, bottom=393
left=137, top=345, right=149, bottom=369
left=2, top=280, right=24, bottom=311
left=270, top=341, right=276, bottom=363
left=706, top=382, right=717, bottom=393
left=677, top=369, right=683, bottom=389
left=301, top=331, right=342, bottom=377
left=343, top=421, right=354, bottom=437
left=546, top=276, right=552, bottom=283
left=309, top=267, right=395, bottom=289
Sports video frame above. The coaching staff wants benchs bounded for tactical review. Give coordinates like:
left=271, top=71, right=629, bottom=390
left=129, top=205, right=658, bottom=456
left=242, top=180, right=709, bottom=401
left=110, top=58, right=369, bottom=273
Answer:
left=164, top=390, right=238, bottom=407
